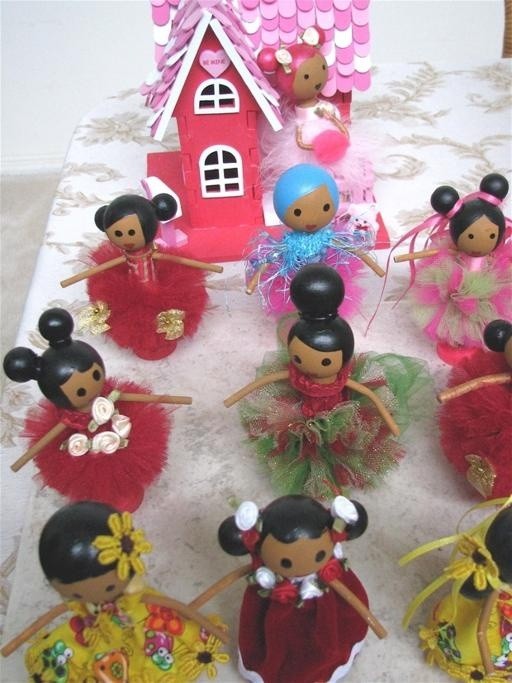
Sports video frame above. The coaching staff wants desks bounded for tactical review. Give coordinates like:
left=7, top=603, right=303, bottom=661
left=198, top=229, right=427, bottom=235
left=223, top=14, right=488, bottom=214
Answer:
left=1, top=59, right=511, bottom=683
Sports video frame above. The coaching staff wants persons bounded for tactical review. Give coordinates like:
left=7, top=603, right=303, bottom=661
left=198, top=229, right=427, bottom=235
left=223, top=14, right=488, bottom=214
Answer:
left=257, top=44, right=378, bottom=204
left=436, top=319, right=512, bottom=499
left=243, top=163, right=386, bottom=324
left=397, top=490, right=512, bottom=683
left=224, top=263, right=429, bottom=504
left=191, top=493, right=388, bottom=681
left=364, top=172, right=512, bottom=348
left=2, top=307, right=193, bottom=513
left=1, top=500, right=228, bottom=682
left=58, top=195, right=223, bottom=360
